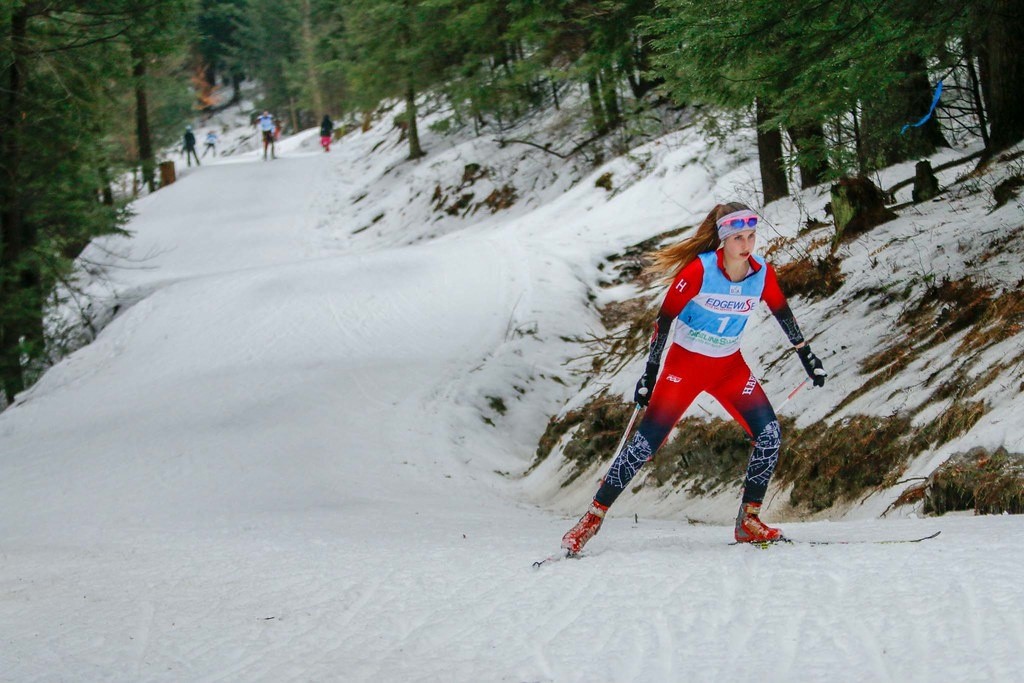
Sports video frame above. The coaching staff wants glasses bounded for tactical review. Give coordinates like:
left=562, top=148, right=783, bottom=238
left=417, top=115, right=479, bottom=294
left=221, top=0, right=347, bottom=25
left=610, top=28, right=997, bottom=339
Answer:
left=721, top=215, right=758, bottom=230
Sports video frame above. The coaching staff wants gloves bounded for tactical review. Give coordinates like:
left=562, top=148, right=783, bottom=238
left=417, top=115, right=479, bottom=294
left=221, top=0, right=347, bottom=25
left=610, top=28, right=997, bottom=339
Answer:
left=634, top=362, right=660, bottom=408
left=796, top=345, right=828, bottom=387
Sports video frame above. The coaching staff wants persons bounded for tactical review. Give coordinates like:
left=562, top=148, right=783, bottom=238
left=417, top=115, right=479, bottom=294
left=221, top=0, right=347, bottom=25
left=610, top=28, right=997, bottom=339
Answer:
left=254, top=111, right=277, bottom=159
left=202, top=131, right=219, bottom=156
left=561, top=202, right=825, bottom=557
left=321, top=114, right=333, bottom=151
left=183, top=126, right=200, bottom=167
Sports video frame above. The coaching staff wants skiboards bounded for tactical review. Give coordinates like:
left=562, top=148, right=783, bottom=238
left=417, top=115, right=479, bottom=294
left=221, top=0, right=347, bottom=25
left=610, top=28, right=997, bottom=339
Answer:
left=531, top=528, right=942, bottom=572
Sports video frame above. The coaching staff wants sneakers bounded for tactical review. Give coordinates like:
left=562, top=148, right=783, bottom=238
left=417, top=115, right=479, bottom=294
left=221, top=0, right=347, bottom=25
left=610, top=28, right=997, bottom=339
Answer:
left=734, top=502, right=783, bottom=543
left=560, top=499, right=608, bottom=558
